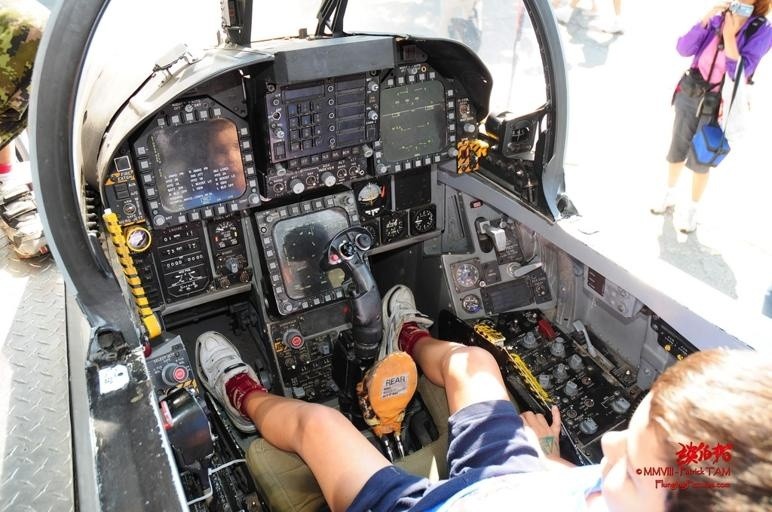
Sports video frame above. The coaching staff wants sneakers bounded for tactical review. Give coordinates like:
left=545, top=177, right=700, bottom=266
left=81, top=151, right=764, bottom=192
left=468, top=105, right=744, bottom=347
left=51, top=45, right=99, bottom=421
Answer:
left=651, top=196, right=674, bottom=215
left=194, top=331, right=264, bottom=434
left=377, top=285, right=434, bottom=362
left=679, top=209, right=702, bottom=233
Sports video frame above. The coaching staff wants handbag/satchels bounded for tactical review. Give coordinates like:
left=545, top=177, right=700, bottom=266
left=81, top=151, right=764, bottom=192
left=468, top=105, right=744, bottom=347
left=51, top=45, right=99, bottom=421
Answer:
left=692, top=124, right=731, bottom=167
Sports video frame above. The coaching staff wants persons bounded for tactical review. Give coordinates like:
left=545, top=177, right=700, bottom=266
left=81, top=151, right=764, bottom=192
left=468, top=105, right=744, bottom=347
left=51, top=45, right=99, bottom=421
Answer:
left=450, top=0, right=483, bottom=51
left=193, top=285, right=772, bottom=512
left=646, top=0, right=772, bottom=235
left=0, top=0, right=50, bottom=267
left=184, top=122, right=246, bottom=209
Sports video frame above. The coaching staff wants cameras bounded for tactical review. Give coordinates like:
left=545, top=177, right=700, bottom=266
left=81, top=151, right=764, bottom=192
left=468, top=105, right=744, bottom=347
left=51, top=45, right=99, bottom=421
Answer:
left=729, top=2, right=754, bottom=18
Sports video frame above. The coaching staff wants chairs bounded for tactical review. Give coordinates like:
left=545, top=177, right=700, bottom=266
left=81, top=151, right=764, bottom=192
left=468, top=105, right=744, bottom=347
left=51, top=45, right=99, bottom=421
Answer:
left=242, top=369, right=450, bottom=512
